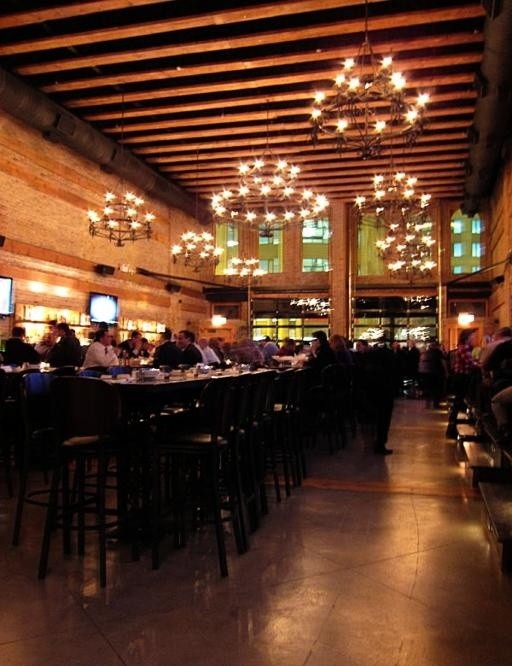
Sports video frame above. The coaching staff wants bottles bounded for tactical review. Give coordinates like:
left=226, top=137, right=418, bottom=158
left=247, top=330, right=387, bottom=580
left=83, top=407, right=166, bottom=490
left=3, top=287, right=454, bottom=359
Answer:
left=119, top=350, right=140, bottom=367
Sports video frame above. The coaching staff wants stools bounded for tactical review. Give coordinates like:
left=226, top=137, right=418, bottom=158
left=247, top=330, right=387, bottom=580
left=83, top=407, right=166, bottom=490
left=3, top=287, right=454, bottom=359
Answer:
left=235, top=361, right=366, bottom=536
left=137, top=377, right=245, bottom=577
left=10, top=367, right=78, bottom=547
left=37, top=378, right=133, bottom=587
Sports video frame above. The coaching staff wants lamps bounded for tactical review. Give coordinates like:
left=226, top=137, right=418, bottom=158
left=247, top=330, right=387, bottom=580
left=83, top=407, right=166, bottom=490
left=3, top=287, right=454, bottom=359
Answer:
left=376, top=230, right=435, bottom=275
left=169, top=151, right=223, bottom=274
left=209, top=100, right=329, bottom=227
left=224, top=229, right=267, bottom=276
left=354, top=156, right=430, bottom=231
left=308, top=4, right=431, bottom=160
left=89, top=91, right=153, bottom=245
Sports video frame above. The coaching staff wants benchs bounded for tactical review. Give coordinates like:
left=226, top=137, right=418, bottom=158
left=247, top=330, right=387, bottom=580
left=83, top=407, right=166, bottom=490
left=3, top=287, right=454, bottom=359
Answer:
left=449, top=393, right=511, bottom=576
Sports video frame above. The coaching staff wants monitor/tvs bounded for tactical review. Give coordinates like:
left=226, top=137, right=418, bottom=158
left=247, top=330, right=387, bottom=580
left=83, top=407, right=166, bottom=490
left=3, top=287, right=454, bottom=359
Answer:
left=89, top=292, right=118, bottom=322
left=0, top=276, right=12, bottom=317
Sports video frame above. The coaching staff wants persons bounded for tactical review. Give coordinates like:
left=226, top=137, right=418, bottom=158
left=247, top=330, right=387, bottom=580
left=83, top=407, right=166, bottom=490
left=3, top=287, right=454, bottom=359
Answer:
left=390, top=337, right=449, bottom=407
left=82, top=329, right=119, bottom=370
left=296, top=330, right=369, bottom=369
left=358, top=338, right=401, bottom=454
left=445, top=327, right=510, bottom=440
left=1, top=326, right=41, bottom=366
left=117, top=331, right=149, bottom=359
left=97, top=322, right=116, bottom=347
left=150, top=328, right=297, bottom=369
left=35, top=320, right=85, bottom=366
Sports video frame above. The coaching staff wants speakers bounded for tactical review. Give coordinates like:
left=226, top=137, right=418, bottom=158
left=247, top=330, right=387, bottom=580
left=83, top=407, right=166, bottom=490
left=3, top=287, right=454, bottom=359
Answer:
left=0, top=235, right=5, bottom=247
left=96, top=264, right=114, bottom=275
left=166, top=283, right=181, bottom=292
left=495, top=276, right=504, bottom=283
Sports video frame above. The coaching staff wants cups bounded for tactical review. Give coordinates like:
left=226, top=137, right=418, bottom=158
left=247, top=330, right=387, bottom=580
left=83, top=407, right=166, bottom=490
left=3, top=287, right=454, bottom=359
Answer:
left=130, top=360, right=250, bottom=384
left=39, top=361, right=51, bottom=373
